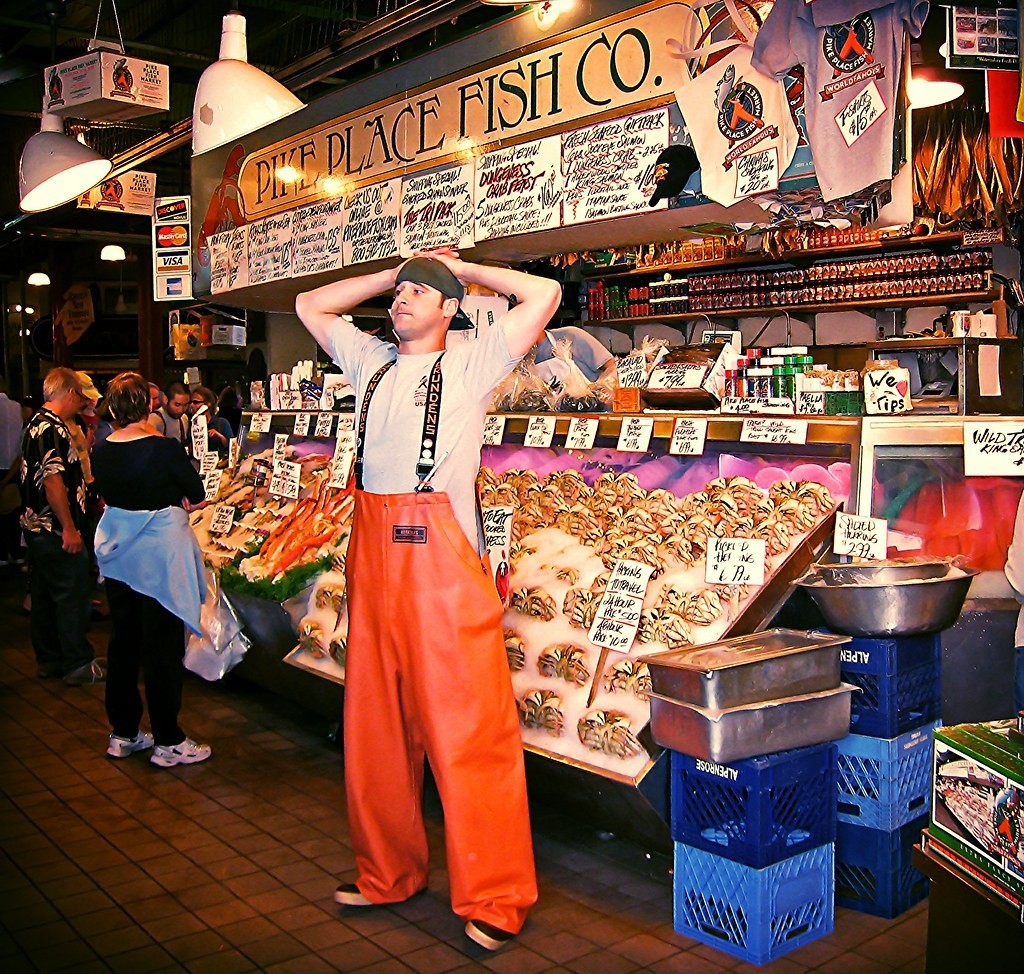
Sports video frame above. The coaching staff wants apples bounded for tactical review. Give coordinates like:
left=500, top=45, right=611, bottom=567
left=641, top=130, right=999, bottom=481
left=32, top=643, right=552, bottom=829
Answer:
left=915, top=224, right=929, bottom=237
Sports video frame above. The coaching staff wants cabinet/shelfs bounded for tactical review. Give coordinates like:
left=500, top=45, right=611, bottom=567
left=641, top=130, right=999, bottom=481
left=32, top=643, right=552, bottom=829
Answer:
left=580, top=226, right=1018, bottom=347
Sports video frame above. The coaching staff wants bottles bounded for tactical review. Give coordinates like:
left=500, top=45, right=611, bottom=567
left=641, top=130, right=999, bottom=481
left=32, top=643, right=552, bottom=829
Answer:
left=724, top=346, right=814, bottom=402
left=858, top=359, right=898, bottom=374
left=586, top=222, right=994, bottom=322
left=269, top=358, right=312, bottom=410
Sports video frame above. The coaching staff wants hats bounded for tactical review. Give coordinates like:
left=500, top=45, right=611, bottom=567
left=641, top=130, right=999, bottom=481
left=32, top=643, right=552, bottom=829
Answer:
left=648, top=146, right=700, bottom=207
left=395, top=257, right=474, bottom=330
left=78, top=372, right=103, bottom=399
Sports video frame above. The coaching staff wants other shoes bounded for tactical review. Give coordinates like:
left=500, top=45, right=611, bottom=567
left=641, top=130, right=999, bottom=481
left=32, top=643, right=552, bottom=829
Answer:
left=465, top=919, right=511, bottom=951
left=65, top=656, right=108, bottom=686
left=38, top=666, right=65, bottom=678
left=333, top=883, right=373, bottom=906
left=91, top=610, right=110, bottom=622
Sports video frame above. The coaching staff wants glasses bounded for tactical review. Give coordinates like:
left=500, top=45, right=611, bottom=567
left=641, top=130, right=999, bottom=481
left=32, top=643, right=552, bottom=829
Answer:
left=75, top=390, right=86, bottom=402
left=188, top=400, right=204, bottom=404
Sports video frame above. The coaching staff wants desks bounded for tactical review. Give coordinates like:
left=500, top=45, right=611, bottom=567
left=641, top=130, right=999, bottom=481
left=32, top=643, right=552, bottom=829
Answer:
left=912, top=842, right=1024, bottom=974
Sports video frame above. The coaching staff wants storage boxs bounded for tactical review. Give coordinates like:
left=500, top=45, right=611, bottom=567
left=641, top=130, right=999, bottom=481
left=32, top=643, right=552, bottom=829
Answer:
left=40, top=49, right=168, bottom=124
left=644, top=626, right=853, bottom=709
left=210, top=323, right=248, bottom=348
left=664, top=745, right=839, bottom=869
left=833, top=720, right=947, bottom=832
left=670, top=839, right=836, bottom=968
left=638, top=688, right=865, bottom=763
left=613, top=386, right=647, bottom=414
left=835, top=810, right=939, bottom=921
left=642, top=344, right=739, bottom=409
left=815, top=629, right=944, bottom=739
left=932, top=718, right=1024, bottom=901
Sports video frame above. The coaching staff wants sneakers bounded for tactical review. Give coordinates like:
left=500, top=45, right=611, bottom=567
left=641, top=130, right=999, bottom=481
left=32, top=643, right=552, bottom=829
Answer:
left=107, top=729, right=155, bottom=758
left=150, top=738, right=212, bottom=767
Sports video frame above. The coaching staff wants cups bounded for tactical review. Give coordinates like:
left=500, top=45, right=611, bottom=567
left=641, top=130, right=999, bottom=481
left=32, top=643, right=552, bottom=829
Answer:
left=951, top=311, right=970, bottom=335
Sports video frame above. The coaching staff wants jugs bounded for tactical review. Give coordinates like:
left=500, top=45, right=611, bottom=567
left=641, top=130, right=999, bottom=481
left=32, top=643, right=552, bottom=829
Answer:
left=932, top=311, right=950, bottom=334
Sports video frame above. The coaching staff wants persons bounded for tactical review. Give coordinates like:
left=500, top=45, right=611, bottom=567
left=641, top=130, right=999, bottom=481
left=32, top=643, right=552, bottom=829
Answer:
left=93, top=400, right=118, bottom=446
left=0, top=377, right=24, bottom=484
left=1002, top=487, right=1024, bottom=710
left=90, top=372, right=211, bottom=767
left=535, top=326, right=615, bottom=382
left=296, top=247, right=562, bottom=950
left=218, top=386, right=244, bottom=435
left=20, top=367, right=106, bottom=686
left=149, top=382, right=234, bottom=457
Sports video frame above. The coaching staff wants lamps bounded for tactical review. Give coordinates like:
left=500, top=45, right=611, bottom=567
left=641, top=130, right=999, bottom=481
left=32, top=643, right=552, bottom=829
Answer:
left=113, top=260, right=129, bottom=314
left=17, top=94, right=114, bottom=215
left=191, top=0, right=310, bottom=158
left=909, top=37, right=968, bottom=110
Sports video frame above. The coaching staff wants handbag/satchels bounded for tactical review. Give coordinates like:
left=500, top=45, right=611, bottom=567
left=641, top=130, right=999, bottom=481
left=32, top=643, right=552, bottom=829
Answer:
left=172, top=313, right=206, bottom=360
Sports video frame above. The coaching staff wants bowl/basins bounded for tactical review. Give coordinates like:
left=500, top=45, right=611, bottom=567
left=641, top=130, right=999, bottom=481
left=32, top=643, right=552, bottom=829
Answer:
left=797, top=565, right=982, bottom=638
left=812, top=561, right=950, bottom=586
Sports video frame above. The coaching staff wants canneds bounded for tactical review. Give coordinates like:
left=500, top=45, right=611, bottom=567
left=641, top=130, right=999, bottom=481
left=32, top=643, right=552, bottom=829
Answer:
left=724, top=346, right=814, bottom=402
left=587, top=278, right=689, bottom=322
left=688, top=246, right=995, bottom=313
